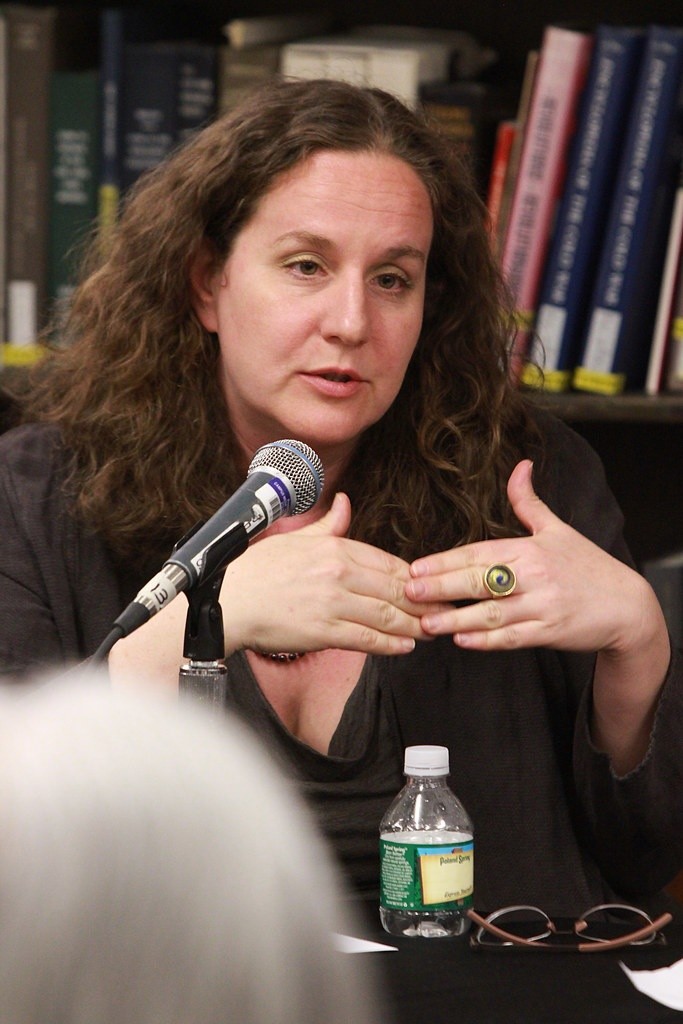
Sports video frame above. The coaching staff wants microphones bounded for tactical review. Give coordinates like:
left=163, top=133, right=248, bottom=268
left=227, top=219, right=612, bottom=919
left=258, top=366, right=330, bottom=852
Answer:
left=112, top=438, right=325, bottom=640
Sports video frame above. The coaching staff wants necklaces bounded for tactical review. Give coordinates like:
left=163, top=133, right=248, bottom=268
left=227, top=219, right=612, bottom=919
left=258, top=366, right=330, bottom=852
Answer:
left=263, top=652, right=304, bottom=663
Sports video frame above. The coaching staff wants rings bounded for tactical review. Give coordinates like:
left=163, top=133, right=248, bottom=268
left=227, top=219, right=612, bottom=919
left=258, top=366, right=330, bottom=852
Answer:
left=485, top=563, right=516, bottom=598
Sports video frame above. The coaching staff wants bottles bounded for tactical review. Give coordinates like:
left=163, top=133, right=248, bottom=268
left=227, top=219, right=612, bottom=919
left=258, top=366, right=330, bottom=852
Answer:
left=378, top=746, right=474, bottom=938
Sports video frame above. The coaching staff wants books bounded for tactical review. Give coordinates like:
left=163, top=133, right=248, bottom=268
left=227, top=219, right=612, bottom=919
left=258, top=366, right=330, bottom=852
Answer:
left=0, top=12, right=683, bottom=416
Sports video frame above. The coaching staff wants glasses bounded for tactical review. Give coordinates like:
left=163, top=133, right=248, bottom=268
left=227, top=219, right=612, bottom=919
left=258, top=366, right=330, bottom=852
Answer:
left=467, top=905, right=672, bottom=951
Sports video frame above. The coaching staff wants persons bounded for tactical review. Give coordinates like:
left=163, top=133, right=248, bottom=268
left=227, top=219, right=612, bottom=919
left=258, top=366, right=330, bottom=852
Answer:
left=0, top=674, right=377, bottom=1023
left=1, top=80, right=683, bottom=918
